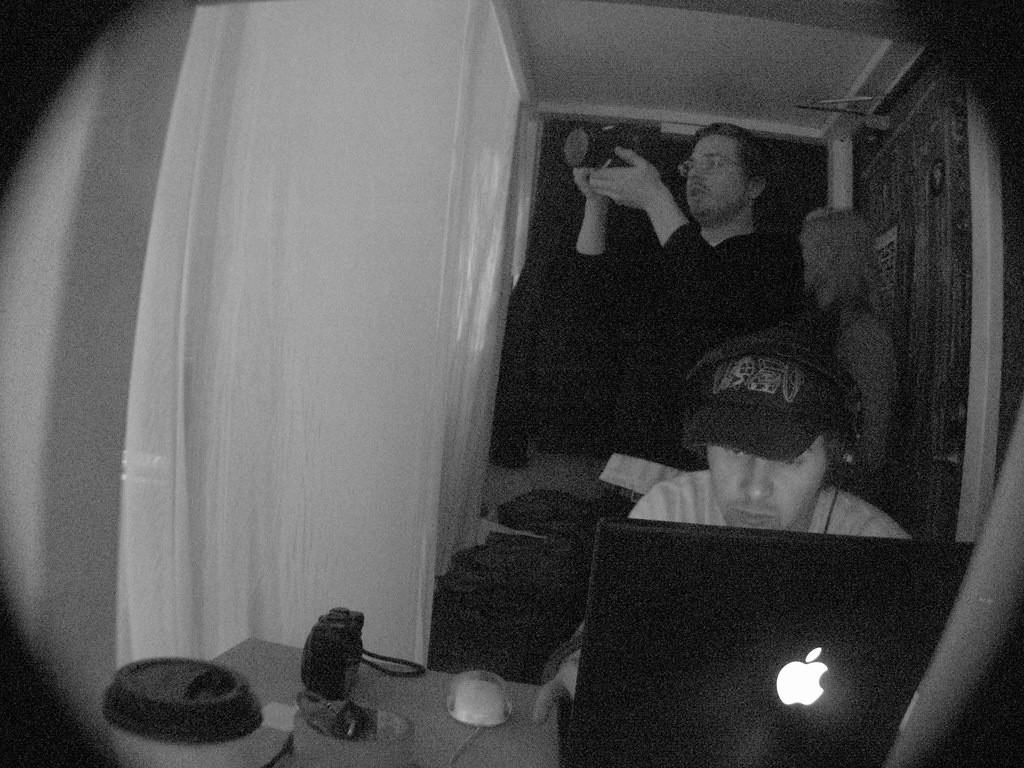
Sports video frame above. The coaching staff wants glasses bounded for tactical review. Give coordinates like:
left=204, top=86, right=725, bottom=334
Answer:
left=677, top=157, right=746, bottom=179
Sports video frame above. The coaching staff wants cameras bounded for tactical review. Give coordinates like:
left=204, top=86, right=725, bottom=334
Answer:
left=301, top=607, right=366, bottom=698
left=564, top=119, right=668, bottom=176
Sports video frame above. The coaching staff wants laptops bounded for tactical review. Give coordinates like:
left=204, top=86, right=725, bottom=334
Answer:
left=560, top=518, right=990, bottom=768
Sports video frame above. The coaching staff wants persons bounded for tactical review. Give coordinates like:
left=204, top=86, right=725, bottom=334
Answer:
left=528, top=346, right=915, bottom=727
left=552, top=121, right=805, bottom=467
left=792, top=204, right=899, bottom=478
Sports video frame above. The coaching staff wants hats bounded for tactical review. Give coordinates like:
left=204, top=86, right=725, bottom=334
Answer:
left=682, top=327, right=847, bottom=462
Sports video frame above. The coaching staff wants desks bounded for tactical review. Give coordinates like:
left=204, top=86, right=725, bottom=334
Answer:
left=202, top=637, right=560, bottom=768
